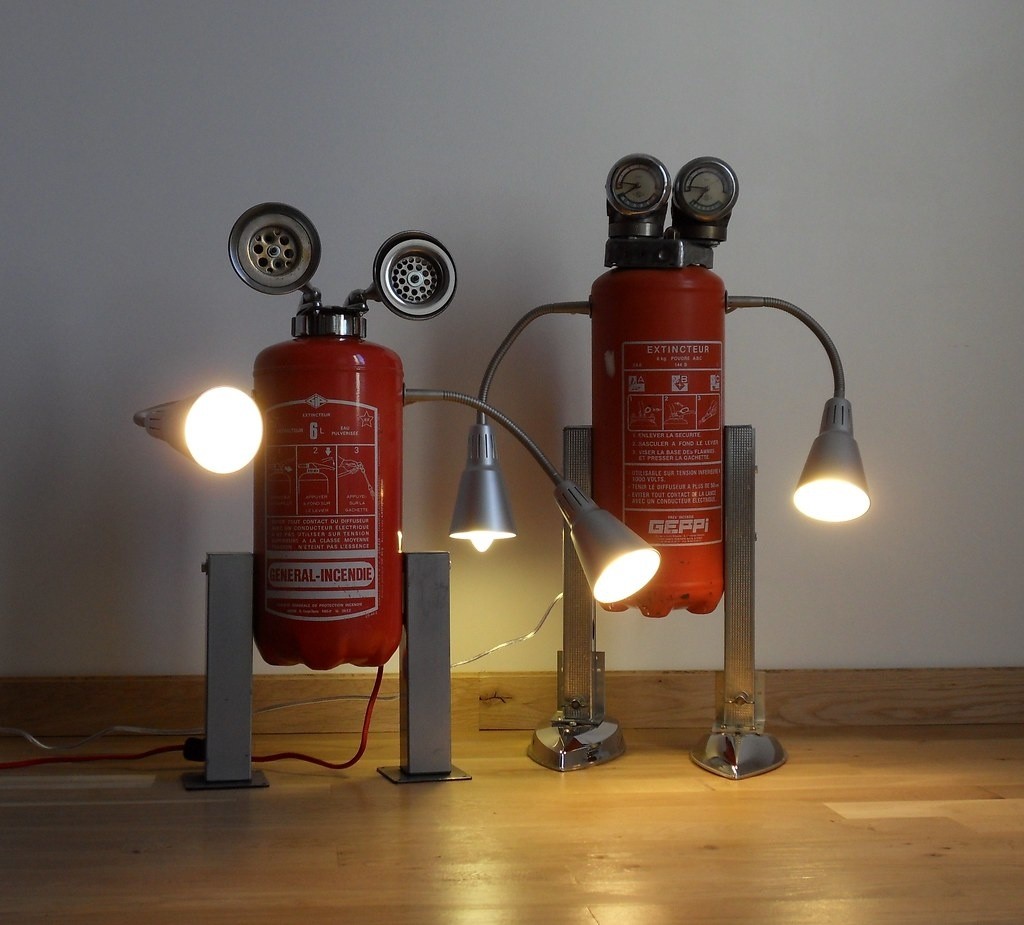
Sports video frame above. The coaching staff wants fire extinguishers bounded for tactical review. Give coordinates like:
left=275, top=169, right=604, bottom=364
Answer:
left=583, top=149, right=741, bottom=621
left=227, top=199, right=461, bottom=670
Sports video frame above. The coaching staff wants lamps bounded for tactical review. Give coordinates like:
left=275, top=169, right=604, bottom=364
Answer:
left=725, top=293, right=870, bottom=522
left=448, top=301, right=590, bottom=553
left=132, top=385, right=263, bottom=475
left=403, top=384, right=661, bottom=604
